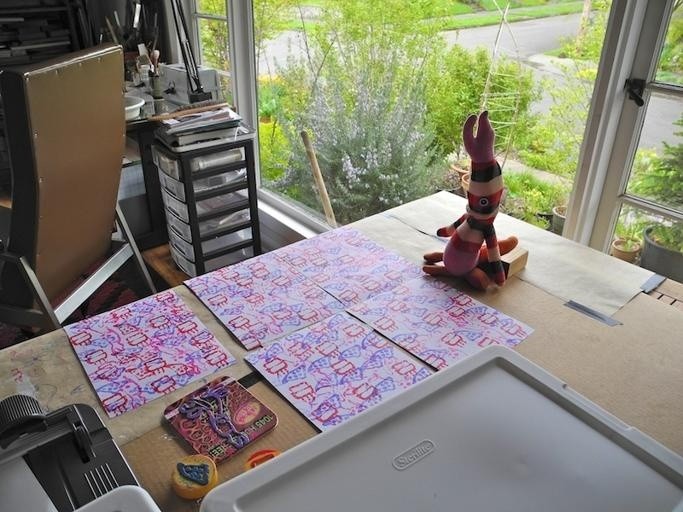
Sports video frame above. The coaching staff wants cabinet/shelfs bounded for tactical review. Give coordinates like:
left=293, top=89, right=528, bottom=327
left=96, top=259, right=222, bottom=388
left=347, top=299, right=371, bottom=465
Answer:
left=142, top=118, right=258, bottom=279
left=0, top=0, right=81, bottom=55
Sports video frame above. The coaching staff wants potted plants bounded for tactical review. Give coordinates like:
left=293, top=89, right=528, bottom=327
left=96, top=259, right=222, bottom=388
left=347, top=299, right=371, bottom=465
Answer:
left=611, top=204, right=683, bottom=285
left=450, top=156, right=568, bottom=237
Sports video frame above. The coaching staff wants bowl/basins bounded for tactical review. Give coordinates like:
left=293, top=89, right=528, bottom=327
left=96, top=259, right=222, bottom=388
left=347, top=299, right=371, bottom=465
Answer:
left=124, top=96, right=146, bottom=121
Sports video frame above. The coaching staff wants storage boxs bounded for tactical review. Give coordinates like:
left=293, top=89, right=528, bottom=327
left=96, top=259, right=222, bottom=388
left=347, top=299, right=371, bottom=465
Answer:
left=160, top=62, right=216, bottom=106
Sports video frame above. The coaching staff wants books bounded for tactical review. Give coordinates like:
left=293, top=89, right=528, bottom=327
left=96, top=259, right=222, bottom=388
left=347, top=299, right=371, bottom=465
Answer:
left=0, top=10, right=74, bottom=70
left=159, top=98, right=242, bottom=146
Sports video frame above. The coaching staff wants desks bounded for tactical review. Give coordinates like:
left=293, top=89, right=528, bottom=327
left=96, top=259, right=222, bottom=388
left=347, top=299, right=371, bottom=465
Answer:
left=119, top=94, right=232, bottom=253
left=1, top=188, right=683, bottom=512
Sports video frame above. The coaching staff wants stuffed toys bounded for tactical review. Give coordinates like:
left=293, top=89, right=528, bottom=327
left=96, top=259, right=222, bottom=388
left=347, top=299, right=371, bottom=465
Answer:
left=423, top=109, right=521, bottom=295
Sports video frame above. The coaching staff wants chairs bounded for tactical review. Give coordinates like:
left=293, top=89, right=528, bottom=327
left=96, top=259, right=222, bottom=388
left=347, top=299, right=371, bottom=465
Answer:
left=0, top=42, right=158, bottom=330
